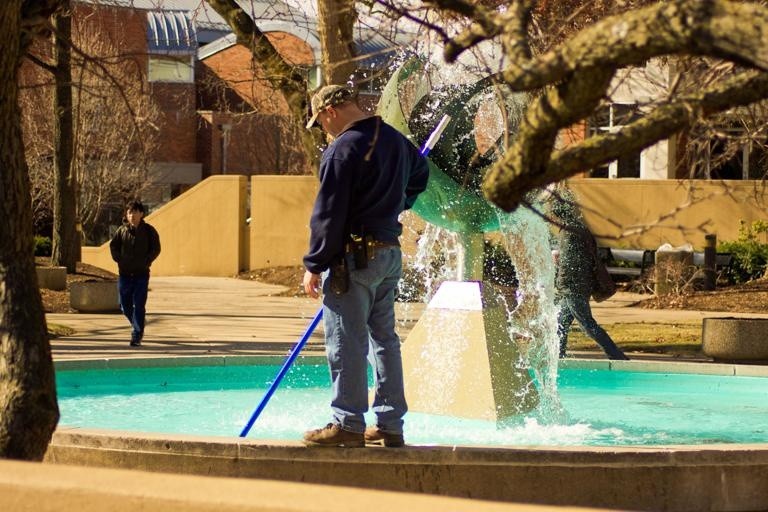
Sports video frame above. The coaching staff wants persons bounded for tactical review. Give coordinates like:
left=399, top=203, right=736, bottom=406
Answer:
left=303, top=84, right=430, bottom=448
left=110, top=200, right=161, bottom=346
left=549, top=187, right=629, bottom=359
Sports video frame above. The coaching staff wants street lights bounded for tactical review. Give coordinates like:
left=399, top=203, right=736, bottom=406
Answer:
left=591, top=264, right=617, bottom=303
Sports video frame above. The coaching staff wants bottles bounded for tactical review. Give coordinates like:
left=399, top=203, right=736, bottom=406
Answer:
left=305, top=85, right=351, bottom=130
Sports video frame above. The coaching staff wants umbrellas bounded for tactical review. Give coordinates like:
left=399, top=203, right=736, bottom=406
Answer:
left=364, top=426, right=404, bottom=448
left=301, top=423, right=365, bottom=449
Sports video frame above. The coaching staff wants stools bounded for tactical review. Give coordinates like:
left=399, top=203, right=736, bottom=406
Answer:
left=345, top=242, right=389, bottom=255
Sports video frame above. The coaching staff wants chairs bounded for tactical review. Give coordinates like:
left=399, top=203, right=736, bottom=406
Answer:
left=130, top=340, right=140, bottom=347
